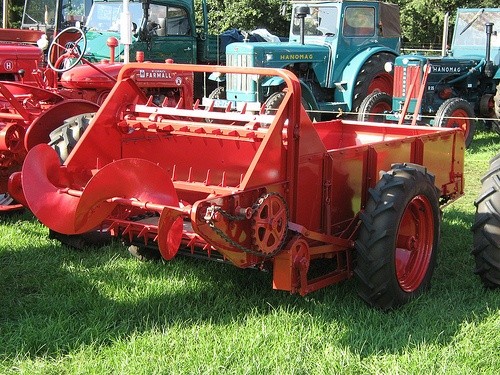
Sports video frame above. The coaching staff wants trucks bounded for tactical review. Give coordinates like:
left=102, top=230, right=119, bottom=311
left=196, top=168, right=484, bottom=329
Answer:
left=62, top=0, right=290, bottom=103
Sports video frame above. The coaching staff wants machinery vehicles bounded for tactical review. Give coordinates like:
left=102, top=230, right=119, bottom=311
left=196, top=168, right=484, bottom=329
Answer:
left=357, top=7, right=500, bottom=150
left=200, top=0, right=425, bottom=128
